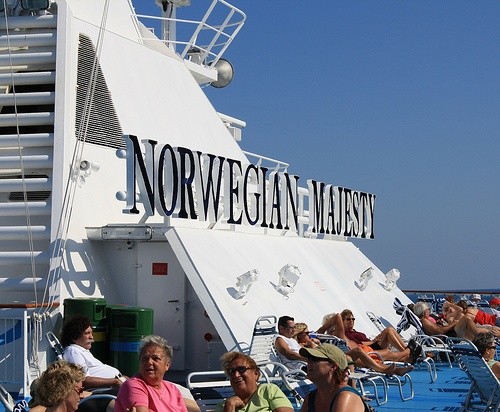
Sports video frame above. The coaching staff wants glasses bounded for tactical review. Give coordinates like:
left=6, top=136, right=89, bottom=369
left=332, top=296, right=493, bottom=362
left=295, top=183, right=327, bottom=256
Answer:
left=226, top=366, right=253, bottom=376
left=345, top=318, right=355, bottom=321
left=75, top=385, right=85, bottom=393
left=303, top=331, right=309, bottom=335
left=490, top=344, right=496, bottom=350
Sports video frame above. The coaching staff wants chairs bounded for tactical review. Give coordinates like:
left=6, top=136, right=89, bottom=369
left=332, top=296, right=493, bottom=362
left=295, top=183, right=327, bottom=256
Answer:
left=0, top=290, right=500, bottom=412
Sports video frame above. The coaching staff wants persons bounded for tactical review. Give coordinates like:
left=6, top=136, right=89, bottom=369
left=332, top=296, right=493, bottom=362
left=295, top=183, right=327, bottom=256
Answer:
left=114, top=336, right=201, bottom=412
left=299, top=343, right=369, bottom=412
left=407, top=294, right=500, bottom=344
left=216, top=351, right=294, bottom=412
left=274, top=316, right=372, bottom=401
left=58, top=317, right=129, bottom=396
left=472, top=332, right=500, bottom=381
left=314, top=309, right=434, bottom=377
left=31, top=361, right=85, bottom=412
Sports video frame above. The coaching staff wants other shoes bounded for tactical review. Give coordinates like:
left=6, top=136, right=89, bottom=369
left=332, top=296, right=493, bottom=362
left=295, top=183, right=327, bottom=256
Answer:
left=405, top=339, right=422, bottom=365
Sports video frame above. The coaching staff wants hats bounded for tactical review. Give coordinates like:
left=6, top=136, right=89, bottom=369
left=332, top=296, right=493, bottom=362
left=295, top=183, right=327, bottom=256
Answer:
left=299, top=343, right=348, bottom=372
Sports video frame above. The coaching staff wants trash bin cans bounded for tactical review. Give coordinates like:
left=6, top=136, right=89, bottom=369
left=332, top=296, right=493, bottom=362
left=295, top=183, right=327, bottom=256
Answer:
left=64, top=298, right=109, bottom=365
left=110, top=305, right=154, bottom=377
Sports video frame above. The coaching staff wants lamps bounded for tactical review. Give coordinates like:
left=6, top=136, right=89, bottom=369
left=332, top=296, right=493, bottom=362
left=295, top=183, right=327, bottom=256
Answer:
left=234, top=268, right=260, bottom=300
left=276, top=262, right=302, bottom=296
left=357, top=266, right=377, bottom=291
left=384, top=267, right=401, bottom=291
left=20, top=0, right=52, bottom=16
